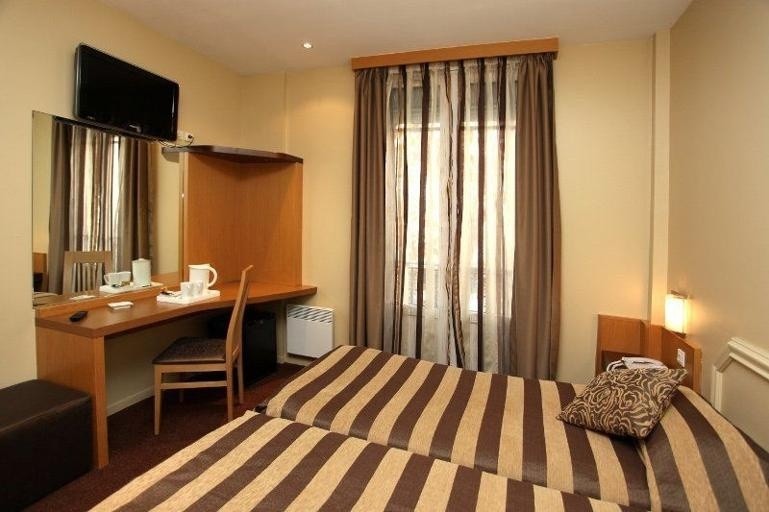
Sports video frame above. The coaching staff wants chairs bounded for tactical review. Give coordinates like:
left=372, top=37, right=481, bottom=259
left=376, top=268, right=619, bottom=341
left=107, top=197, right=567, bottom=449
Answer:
left=152, top=262, right=255, bottom=436
left=63, top=251, right=114, bottom=295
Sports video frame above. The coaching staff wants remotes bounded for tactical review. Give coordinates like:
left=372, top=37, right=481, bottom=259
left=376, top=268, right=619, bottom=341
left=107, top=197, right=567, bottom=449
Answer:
left=70, top=311, right=87, bottom=321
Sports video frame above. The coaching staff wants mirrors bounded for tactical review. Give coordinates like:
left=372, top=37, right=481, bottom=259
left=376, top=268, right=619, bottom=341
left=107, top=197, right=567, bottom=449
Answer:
left=31, top=109, right=183, bottom=311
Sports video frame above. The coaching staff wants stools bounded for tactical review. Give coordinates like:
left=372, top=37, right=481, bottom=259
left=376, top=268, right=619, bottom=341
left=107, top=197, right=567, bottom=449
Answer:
left=1, top=378, right=92, bottom=511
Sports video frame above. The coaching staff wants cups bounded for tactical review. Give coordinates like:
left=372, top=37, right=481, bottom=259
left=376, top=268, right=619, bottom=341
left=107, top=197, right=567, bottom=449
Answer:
left=104, top=273, right=118, bottom=284
left=119, top=272, right=131, bottom=282
left=191, top=281, right=202, bottom=295
left=180, top=282, right=191, bottom=297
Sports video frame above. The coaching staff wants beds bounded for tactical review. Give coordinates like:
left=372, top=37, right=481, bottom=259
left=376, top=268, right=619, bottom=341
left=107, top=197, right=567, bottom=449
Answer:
left=89, top=335, right=769, bottom=512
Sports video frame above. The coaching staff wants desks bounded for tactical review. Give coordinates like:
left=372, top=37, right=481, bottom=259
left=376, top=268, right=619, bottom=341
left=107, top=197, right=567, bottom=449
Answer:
left=595, top=312, right=703, bottom=396
left=35, top=282, right=319, bottom=472
left=34, top=271, right=179, bottom=309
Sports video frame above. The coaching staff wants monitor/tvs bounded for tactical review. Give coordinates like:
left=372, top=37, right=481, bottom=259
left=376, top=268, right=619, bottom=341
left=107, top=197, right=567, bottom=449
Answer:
left=73, top=42, right=179, bottom=141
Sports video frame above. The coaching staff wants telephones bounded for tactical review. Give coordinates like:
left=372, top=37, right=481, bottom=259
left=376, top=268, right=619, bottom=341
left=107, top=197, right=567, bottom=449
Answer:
left=621, top=356, right=666, bottom=368
